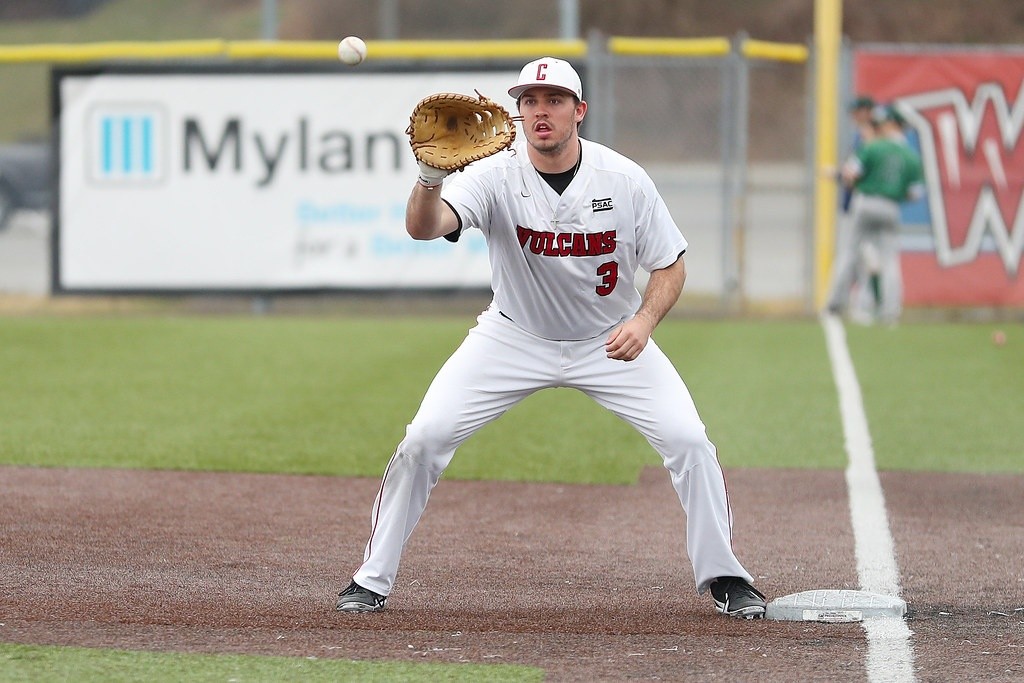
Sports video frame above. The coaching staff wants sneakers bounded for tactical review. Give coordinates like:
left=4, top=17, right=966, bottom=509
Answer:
left=710, top=576, right=766, bottom=619
left=336, top=579, right=387, bottom=611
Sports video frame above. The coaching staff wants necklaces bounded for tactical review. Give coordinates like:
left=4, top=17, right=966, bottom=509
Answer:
left=531, top=139, right=581, bottom=230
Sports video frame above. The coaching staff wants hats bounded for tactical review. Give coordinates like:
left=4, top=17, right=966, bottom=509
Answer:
left=507, top=56, right=582, bottom=102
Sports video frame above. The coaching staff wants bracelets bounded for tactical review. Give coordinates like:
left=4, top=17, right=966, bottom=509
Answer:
left=418, top=178, right=443, bottom=191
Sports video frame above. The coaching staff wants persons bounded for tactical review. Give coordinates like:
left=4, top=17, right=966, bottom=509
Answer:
left=826, top=104, right=925, bottom=327
left=823, top=99, right=885, bottom=325
left=333, top=56, right=768, bottom=620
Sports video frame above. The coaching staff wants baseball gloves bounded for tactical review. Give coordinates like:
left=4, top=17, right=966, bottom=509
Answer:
left=404, top=85, right=528, bottom=175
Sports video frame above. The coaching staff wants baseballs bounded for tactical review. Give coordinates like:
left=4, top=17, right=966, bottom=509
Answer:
left=335, top=34, right=368, bottom=67
